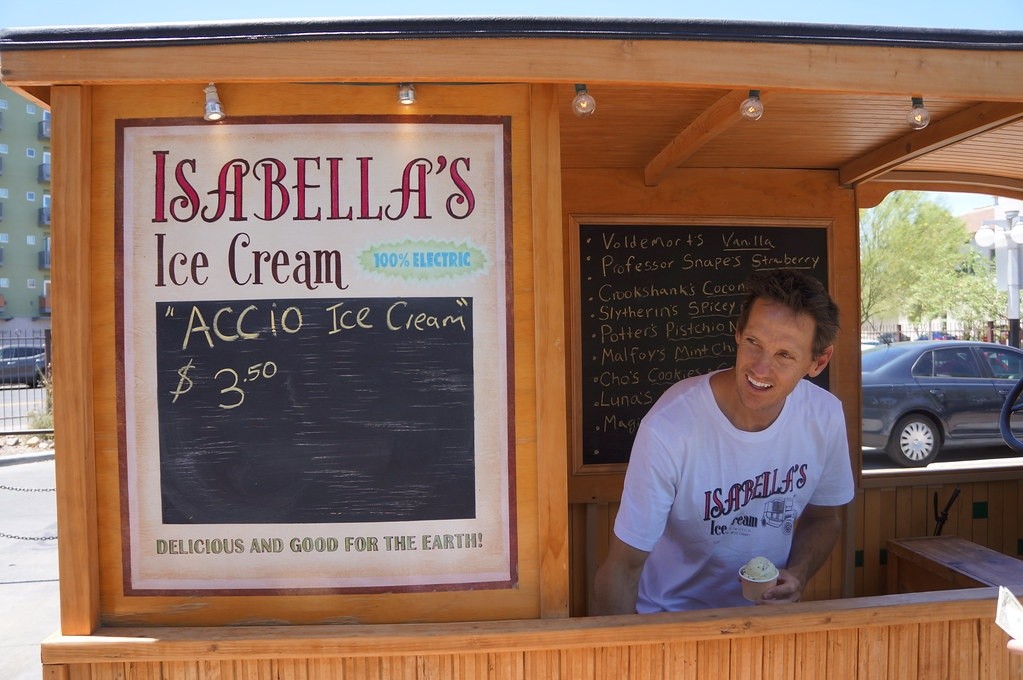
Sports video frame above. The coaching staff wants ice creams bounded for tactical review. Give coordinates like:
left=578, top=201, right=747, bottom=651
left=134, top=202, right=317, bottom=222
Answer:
left=742, top=557, right=778, bottom=581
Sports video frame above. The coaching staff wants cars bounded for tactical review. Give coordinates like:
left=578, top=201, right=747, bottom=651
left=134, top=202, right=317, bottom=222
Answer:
left=1, top=344, right=49, bottom=389
left=860, top=340, right=1023, bottom=468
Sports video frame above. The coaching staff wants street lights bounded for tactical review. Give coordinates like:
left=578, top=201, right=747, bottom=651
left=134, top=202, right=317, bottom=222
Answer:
left=973, top=196, right=1023, bottom=348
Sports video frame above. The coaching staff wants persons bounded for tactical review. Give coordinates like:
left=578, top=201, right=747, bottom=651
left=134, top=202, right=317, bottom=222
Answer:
left=591, top=268, right=857, bottom=616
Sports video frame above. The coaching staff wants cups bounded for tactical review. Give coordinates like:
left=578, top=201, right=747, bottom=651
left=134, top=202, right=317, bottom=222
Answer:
left=738, top=564, right=779, bottom=602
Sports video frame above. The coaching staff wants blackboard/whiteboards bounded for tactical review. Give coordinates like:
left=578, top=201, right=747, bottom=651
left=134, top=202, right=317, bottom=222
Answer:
left=568, top=211, right=841, bottom=476
left=152, top=294, right=478, bottom=525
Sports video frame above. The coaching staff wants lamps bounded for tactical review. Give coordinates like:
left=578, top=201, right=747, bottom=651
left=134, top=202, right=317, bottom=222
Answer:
left=200, top=77, right=227, bottom=126
left=393, top=81, right=421, bottom=107
left=901, top=96, right=934, bottom=130
left=738, top=87, right=768, bottom=124
left=568, top=83, right=597, bottom=117
